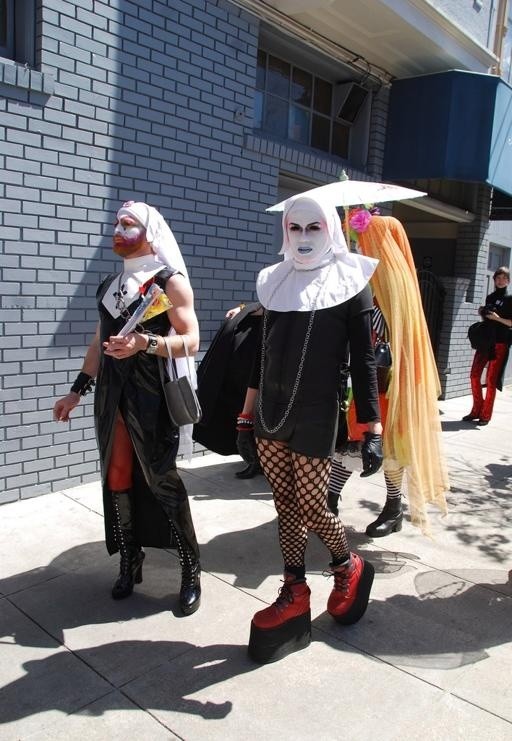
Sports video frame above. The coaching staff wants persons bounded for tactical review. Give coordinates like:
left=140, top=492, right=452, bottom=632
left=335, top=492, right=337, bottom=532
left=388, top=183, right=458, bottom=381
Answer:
left=327, top=207, right=451, bottom=542
left=462, top=266, right=512, bottom=425
left=192, top=300, right=264, bottom=478
left=53, top=201, right=202, bottom=615
left=236, top=192, right=383, bottom=665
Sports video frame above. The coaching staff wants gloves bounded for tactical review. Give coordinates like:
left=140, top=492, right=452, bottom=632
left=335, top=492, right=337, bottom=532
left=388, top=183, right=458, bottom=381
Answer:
left=359, top=428, right=383, bottom=479
left=235, top=414, right=261, bottom=463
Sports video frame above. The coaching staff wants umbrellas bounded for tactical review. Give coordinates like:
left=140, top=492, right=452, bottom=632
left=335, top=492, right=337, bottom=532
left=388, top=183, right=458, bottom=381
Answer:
left=264, top=170, right=429, bottom=251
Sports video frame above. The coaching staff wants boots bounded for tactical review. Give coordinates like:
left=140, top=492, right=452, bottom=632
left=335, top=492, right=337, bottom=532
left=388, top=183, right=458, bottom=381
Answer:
left=324, top=490, right=343, bottom=518
left=164, top=499, right=202, bottom=615
left=108, top=487, right=146, bottom=601
left=365, top=497, right=406, bottom=536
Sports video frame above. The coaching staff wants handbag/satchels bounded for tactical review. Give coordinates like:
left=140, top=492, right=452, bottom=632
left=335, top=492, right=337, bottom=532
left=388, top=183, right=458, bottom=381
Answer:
left=468, top=321, right=494, bottom=350
left=374, top=339, right=392, bottom=396
left=163, top=375, right=203, bottom=430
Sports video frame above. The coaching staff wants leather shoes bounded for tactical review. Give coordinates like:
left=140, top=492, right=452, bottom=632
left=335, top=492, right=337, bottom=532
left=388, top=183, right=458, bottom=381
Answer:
left=460, top=411, right=489, bottom=427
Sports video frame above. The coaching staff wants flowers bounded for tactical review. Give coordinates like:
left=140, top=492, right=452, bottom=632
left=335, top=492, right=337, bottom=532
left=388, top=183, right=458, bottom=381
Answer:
left=339, top=202, right=383, bottom=238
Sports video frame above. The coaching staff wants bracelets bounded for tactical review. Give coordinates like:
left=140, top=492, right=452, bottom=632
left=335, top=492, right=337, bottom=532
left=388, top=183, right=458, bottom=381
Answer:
left=239, top=303, right=246, bottom=310
left=146, top=332, right=159, bottom=354
left=236, top=414, right=256, bottom=431
left=70, top=372, right=95, bottom=397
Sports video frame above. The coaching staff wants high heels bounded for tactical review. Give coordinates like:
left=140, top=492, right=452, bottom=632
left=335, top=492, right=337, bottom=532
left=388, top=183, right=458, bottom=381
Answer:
left=324, top=551, right=379, bottom=626
left=234, top=463, right=262, bottom=480
left=246, top=567, right=315, bottom=665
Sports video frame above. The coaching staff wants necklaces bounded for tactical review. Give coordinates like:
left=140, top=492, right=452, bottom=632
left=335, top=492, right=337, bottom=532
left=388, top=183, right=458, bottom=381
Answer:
left=259, top=266, right=331, bottom=435
left=112, top=282, right=128, bottom=309
